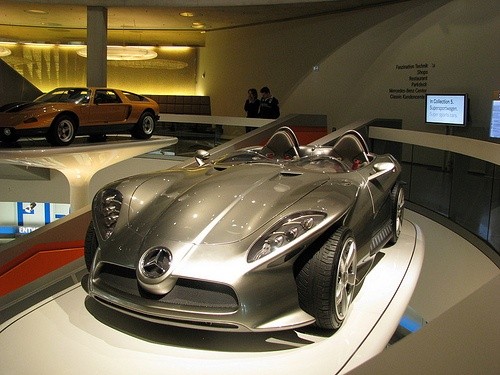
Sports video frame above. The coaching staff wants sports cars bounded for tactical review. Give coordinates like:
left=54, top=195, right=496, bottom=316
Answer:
left=0, top=85, right=161, bottom=146
left=80, top=123, right=409, bottom=334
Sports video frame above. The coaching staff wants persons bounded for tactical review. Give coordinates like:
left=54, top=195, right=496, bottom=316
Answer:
left=244, top=88, right=260, bottom=133
left=258, top=86, right=280, bottom=120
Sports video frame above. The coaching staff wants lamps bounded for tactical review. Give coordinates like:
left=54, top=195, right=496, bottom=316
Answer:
left=0, top=44, right=12, bottom=58
left=76, top=44, right=159, bottom=61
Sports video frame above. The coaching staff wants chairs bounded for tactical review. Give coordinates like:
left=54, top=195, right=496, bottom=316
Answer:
left=258, top=126, right=372, bottom=170
